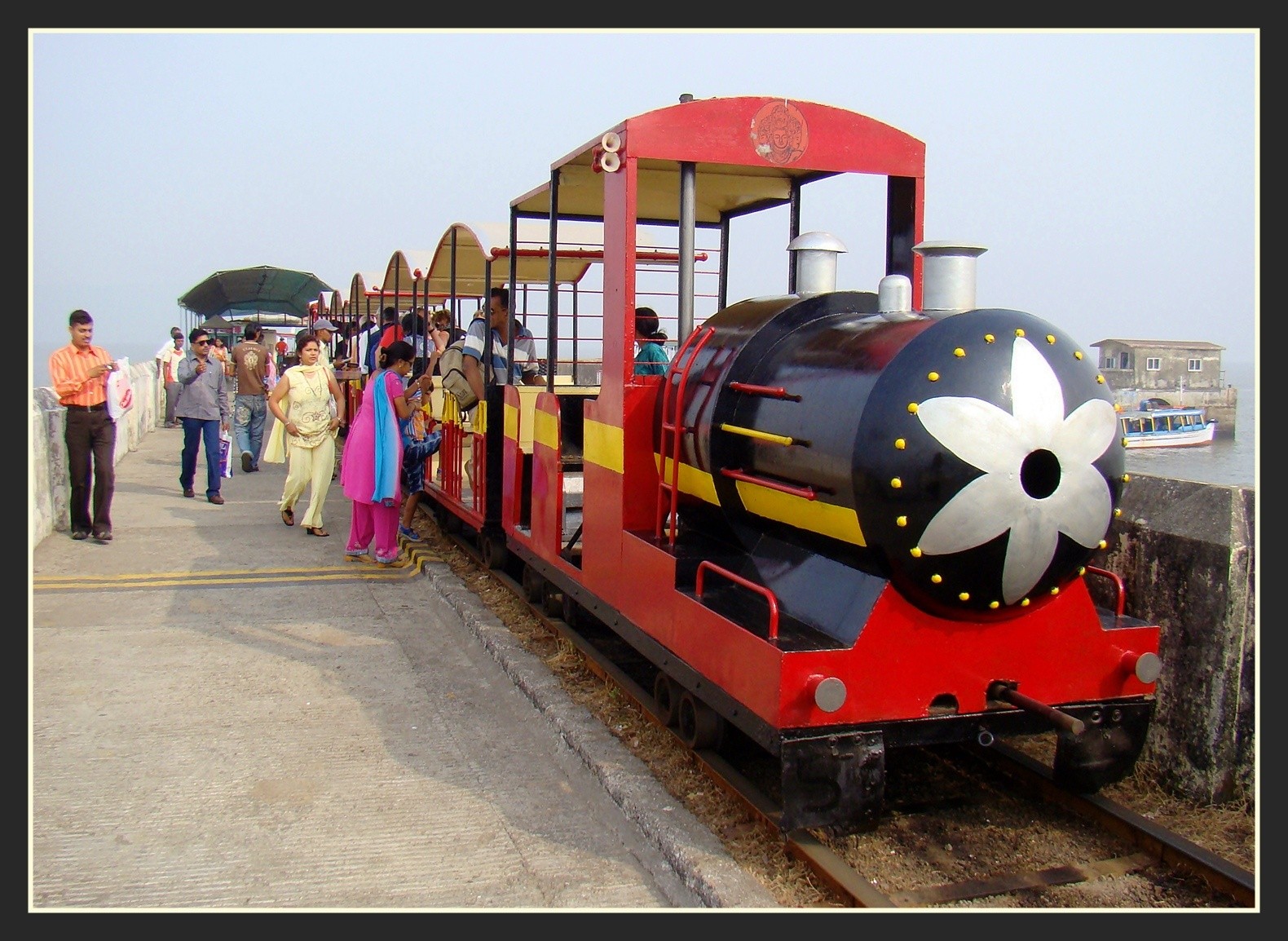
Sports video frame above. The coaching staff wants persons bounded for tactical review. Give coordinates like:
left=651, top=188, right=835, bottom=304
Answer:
left=208, top=334, right=276, bottom=401
left=163, top=332, right=186, bottom=428
left=174, top=328, right=230, bottom=505
left=463, top=287, right=547, bottom=497
left=397, top=374, right=442, bottom=541
left=264, top=336, right=345, bottom=537
left=229, top=322, right=270, bottom=472
left=275, top=338, right=288, bottom=366
left=313, top=319, right=348, bottom=371
left=49, top=310, right=120, bottom=541
left=634, top=308, right=670, bottom=375
left=156, top=327, right=181, bottom=425
left=333, top=306, right=485, bottom=376
left=341, top=341, right=430, bottom=568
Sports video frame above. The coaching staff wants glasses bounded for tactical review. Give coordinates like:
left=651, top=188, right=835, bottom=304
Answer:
left=405, top=360, right=413, bottom=367
left=194, top=340, right=211, bottom=347
left=482, top=302, right=507, bottom=316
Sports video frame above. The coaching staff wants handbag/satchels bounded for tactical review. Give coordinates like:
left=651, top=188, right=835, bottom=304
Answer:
left=218, top=430, right=233, bottom=479
left=107, top=357, right=134, bottom=421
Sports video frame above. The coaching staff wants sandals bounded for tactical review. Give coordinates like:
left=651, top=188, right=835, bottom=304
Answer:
left=398, top=525, right=423, bottom=542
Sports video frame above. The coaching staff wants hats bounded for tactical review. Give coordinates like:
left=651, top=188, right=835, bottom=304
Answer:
left=359, top=315, right=377, bottom=329
left=313, top=320, right=340, bottom=332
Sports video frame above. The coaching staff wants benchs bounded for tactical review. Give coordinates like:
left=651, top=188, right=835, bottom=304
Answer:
left=427, top=375, right=600, bottom=529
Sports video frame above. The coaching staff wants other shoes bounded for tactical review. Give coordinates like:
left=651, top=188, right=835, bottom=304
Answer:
left=208, top=494, right=224, bottom=504
left=165, top=423, right=176, bottom=428
left=266, top=392, right=272, bottom=400
left=94, top=530, right=114, bottom=541
left=184, top=487, right=194, bottom=497
left=72, top=531, right=88, bottom=539
left=241, top=453, right=253, bottom=473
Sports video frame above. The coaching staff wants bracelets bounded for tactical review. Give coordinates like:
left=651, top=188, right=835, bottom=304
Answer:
left=285, top=421, right=291, bottom=426
left=532, top=374, right=542, bottom=382
left=335, top=416, right=342, bottom=422
left=428, top=328, right=435, bottom=334
left=415, top=379, right=420, bottom=388
left=407, top=401, right=417, bottom=407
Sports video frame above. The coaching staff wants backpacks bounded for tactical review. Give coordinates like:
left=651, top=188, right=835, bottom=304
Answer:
left=439, top=317, right=496, bottom=411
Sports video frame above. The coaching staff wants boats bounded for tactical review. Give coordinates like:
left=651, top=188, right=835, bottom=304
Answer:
left=1120, top=406, right=1218, bottom=449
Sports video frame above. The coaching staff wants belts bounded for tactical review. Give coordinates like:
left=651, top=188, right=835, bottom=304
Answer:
left=68, top=402, right=107, bottom=413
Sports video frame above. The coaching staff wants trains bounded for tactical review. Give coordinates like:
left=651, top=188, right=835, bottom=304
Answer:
left=311, top=94, right=1161, bottom=834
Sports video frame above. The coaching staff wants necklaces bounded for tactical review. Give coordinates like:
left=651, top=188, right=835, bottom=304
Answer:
left=300, top=368, right=323, bottom=401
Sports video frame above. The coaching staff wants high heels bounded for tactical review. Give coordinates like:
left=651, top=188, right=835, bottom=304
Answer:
left=378, top=559, right=404, bottom=568
left=307, top=527, right=330, bottom=537
left=282, top=505, right=295, bottom=525
left=346, top=555, right=377, bottom=563
